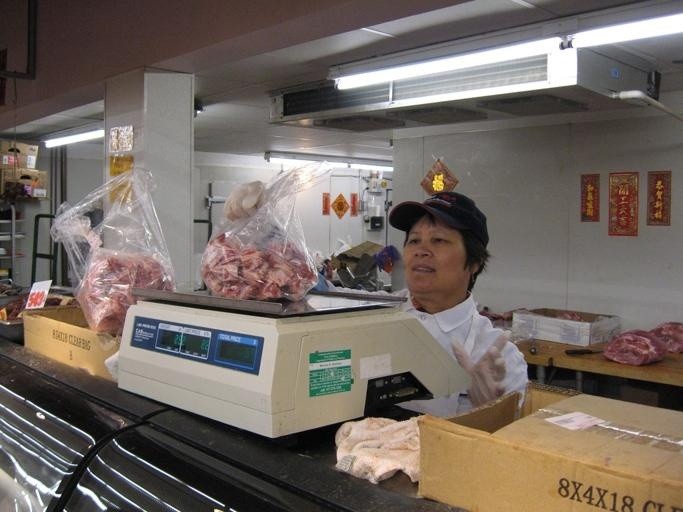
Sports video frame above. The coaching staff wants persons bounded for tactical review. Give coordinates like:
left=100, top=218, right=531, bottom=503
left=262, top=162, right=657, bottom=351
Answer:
left=311, top=192, right=528, bottom=421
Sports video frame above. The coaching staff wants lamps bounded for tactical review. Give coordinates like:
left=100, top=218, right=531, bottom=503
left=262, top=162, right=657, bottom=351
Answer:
left=327, top=0, right=683, bottom=91
left=40, top=99, right=203, bottom=148
left=264, top=151, right=393, bottom=172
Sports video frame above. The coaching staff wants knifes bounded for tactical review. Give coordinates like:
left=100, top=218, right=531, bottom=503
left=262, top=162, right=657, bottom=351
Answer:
left=565, top=346, right=604, bottom=355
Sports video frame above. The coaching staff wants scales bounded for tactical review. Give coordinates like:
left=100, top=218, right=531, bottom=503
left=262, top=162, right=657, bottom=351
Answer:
left=118, top=288, right=473, bottom=438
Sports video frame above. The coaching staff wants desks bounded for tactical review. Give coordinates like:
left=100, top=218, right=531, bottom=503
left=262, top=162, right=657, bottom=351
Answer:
left=552, top=342, right=683, bottom=392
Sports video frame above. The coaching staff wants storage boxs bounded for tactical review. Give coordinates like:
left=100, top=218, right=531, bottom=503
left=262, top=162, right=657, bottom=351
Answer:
left=1, top=168, right=48, bottom=198
left=17, top=304, right=122, bottom=379
left=411, top=371, right=683, bottom=511
left=0, top=141, right=39, bottom=170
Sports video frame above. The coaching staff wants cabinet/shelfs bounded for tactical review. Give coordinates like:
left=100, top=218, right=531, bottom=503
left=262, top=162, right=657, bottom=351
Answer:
left=0, top=340, right=470, bottom=512
left=0, top=199, right=26, bottom=282
left=511, top=337, right=567, bottom=384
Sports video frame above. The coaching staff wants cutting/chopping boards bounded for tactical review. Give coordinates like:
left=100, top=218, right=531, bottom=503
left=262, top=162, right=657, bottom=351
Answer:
left=512, top=335, right=683, bottom=388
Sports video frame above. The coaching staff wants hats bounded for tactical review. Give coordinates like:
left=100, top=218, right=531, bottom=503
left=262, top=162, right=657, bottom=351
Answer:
left=385, top=189, right=488, bottom=247
